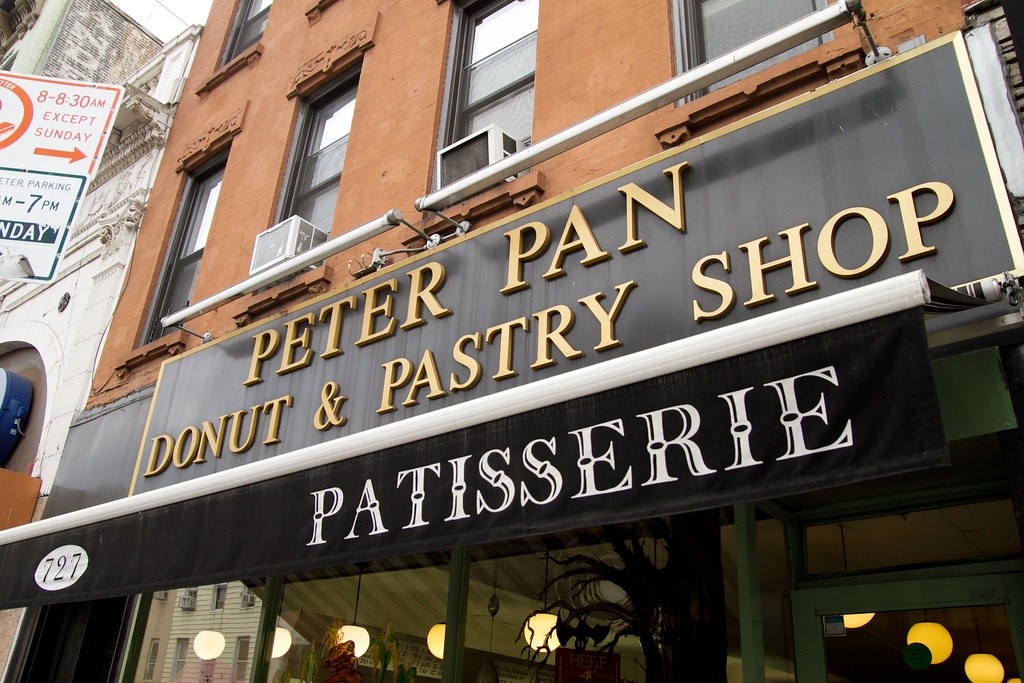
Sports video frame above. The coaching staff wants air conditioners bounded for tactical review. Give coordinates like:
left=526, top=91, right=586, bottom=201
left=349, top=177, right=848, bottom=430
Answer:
left=249, top=215, right=328, bottom=287
left=435, top=123, right=530, bottom=192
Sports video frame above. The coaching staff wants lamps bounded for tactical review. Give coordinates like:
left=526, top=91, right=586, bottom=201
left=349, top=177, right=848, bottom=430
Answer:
left=1007, top=677, right=1021, bottom=683
left=522, top=550, right=560, bottom=653
left=964, top=618, right=1004, bottom=683
left=193, top=606, right=226, bottom=661
left=427, top=623, right=446, bottom=661
left=836, top=522, right=876, bottom=629
left=335, top=574, right=371, bottom=659
left=271, top=582, right=293, bottom=658
